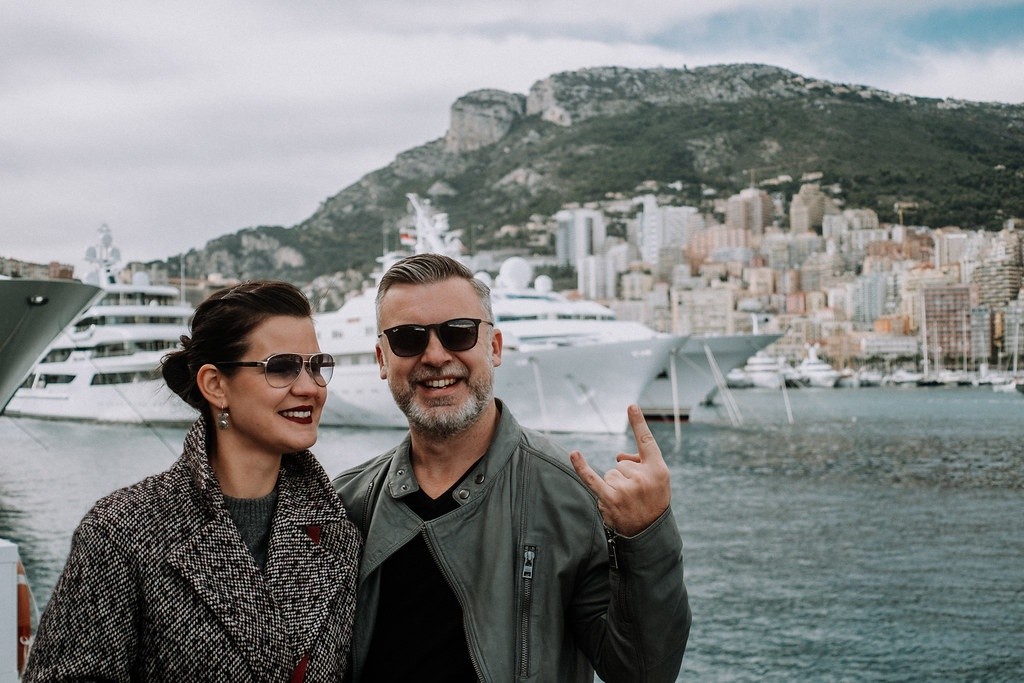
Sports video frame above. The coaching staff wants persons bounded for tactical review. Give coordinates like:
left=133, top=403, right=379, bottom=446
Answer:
left=331, top=253, right=692, bottom=683
left=21, top=280, right=366, bottom=683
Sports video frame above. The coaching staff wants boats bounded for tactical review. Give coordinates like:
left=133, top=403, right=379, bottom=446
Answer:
left=858, top=295, right=1024, bottom=394
left=5, top=201, right=788, bottom=436
left=797, top=343, right=851, bottom=389
left=724, top=311, right=785, bottom=388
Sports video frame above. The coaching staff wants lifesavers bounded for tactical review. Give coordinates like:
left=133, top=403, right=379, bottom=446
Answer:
left=17, top=554, right=33, bottom=679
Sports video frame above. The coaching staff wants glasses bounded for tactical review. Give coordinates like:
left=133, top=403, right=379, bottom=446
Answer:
left=376, top=319, right=495, bottom=358
left=204, top=353, right=335, bottom=389
